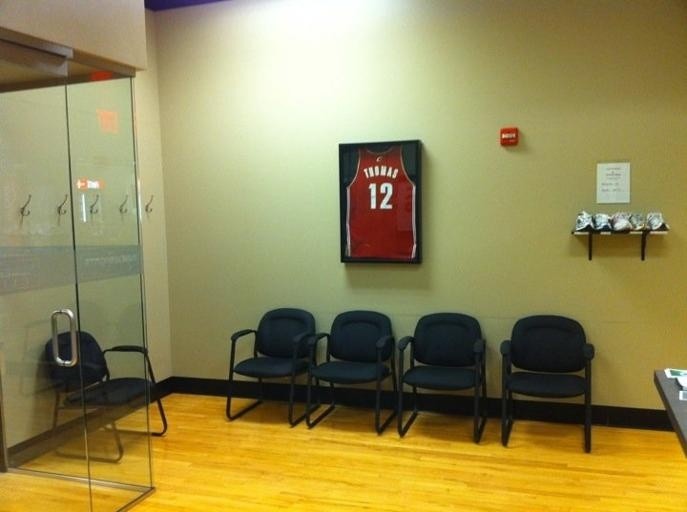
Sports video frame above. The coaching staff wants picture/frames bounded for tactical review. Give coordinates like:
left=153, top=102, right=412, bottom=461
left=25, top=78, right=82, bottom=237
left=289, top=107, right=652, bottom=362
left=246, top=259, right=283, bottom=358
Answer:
left=338, top=138, right=423, bottom=262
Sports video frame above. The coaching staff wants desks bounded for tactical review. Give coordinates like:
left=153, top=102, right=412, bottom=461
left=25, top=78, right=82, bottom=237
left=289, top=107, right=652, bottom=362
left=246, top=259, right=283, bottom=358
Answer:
left=653, top=367, right=686, bottom=457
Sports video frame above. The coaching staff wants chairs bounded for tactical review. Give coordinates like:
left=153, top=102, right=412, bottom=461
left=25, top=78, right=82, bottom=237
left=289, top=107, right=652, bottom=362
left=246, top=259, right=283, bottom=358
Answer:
left=225, top=308, right=322, bottom=426
left=45, top=332, right=167, bottom=462
left=399, top=312, right=488, bottom=443
left=500, top=316, right=596, bottom=453
left=306, top=311, right=399, bottom=433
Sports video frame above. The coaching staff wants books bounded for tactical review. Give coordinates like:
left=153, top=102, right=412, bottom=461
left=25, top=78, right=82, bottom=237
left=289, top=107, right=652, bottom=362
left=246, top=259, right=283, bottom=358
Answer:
left=675, top=375, right=687, bottom=390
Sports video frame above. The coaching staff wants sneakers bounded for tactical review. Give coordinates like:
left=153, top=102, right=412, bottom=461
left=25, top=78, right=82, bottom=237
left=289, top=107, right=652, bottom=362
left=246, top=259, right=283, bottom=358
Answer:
left=575, top=210, right=670, bottom=232
